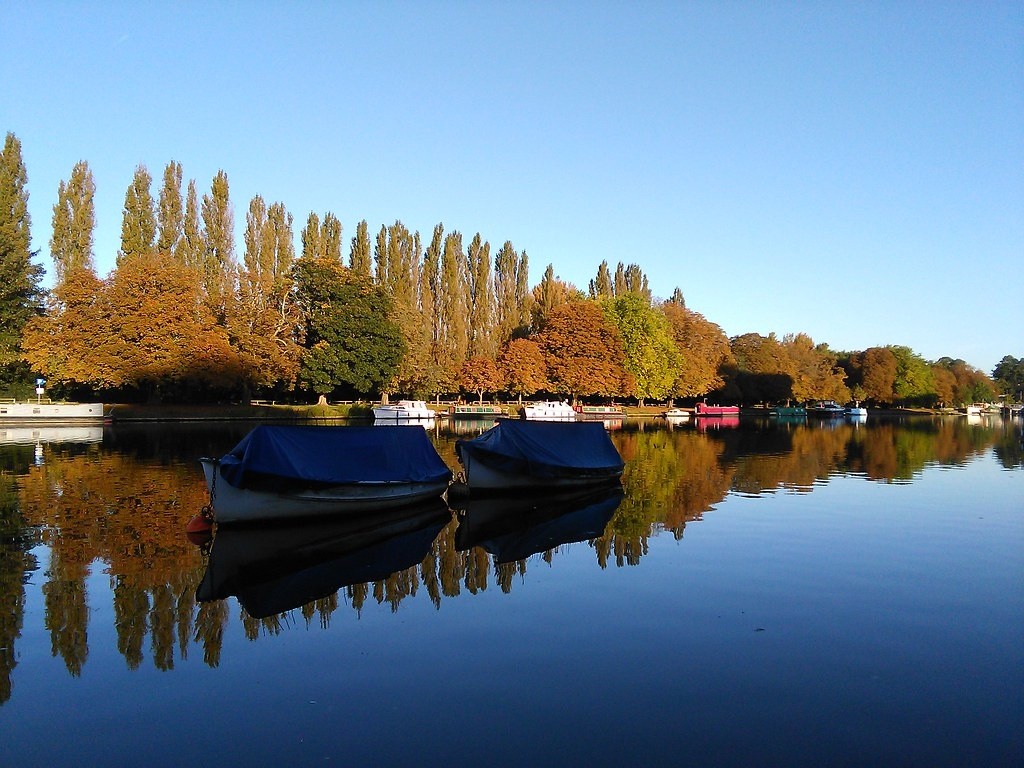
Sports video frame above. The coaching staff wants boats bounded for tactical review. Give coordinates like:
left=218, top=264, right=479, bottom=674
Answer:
left=453, top=416, right=626, bottom=494
left=374, top=419, right=436, bottom=432
left=850, top=401, right=868, bottom=415
left=193, top=494, right=452, bottom=617
left=851, top=415, right=868, bottom=430
left=520, top=400, right=579, bottom=416
left=195, top=421, right=455, bottom=530
left=665, top=409, right=691, bottom=416
left=774, top=407, right=808, bottom=416
left=816, top=400, right=845, bottom=413
left=693, top=402, right=740, bottom=415
left=769, top=412, right=778, bottom=420
left=372, top=400, right=437, bottom=419
left=450, top=483, right=623, bottom=566
